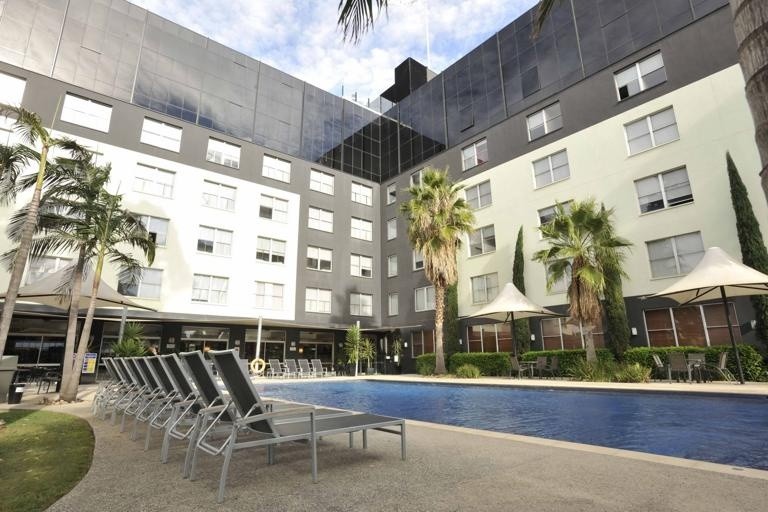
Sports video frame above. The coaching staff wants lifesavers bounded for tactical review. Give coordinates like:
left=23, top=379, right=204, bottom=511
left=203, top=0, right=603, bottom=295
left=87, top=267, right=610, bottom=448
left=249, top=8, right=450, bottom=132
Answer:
left=250, top=358, right=266, bottom=374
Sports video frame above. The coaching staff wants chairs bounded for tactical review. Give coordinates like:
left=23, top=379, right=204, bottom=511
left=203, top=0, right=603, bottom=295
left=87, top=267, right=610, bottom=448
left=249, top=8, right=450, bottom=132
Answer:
left=651, top=351, right=738, bottom=386
left=161, top=348, right=369, bottom=479
left=189, top=347, right=406, bottom=504
left=16, top=366, right=59, bottom=393
left=511, top=356, right=559, bottom=380
left=94, top=348, right=317, bottom=461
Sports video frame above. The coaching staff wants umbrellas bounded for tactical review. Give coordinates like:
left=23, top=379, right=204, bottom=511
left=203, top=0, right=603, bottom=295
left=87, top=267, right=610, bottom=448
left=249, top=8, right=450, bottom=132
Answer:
left=460, top=282, right=563, bottom=356
left=0, top=256, right=158, bottom=391
left=646, top=247, right=768, bottom=383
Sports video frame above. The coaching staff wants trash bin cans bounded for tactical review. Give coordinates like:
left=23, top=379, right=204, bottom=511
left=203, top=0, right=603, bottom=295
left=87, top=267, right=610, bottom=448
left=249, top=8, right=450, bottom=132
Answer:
left=8, top=384, right=25, bottom=404
